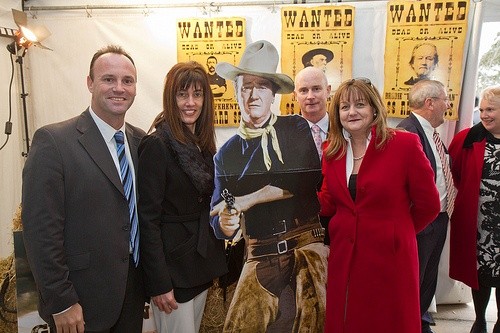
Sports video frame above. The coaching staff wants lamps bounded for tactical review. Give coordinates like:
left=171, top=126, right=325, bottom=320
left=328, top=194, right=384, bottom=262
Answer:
left=0, top=8, right=54, bottom=157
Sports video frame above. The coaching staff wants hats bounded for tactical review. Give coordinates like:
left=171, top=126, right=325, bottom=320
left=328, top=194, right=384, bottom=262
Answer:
left=301, top=48, right=334, bottom=62
left=215, top=40, right=295, bottom=94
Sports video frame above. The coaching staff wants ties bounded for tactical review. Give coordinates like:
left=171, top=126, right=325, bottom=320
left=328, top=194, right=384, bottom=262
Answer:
left=114, top=131, right=140, bottom=269
left=432, top=128, right=456, bottom=219
left=311, top=125, right=323, bottom=161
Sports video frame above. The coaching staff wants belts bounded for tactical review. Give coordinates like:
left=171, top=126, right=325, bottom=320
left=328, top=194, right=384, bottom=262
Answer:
left=247, top=228, right=326, bottom=260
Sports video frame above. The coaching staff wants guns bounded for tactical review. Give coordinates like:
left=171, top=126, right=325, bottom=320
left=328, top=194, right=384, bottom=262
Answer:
left=221, top=188, right=236, bottom=211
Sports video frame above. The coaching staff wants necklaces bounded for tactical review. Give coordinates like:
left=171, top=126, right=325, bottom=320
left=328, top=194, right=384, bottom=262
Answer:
left=354, top=153, right=365, bottom=160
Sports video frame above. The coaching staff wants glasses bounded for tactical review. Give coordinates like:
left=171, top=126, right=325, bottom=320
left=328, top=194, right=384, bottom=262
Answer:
left=431, top=97, right=449, bottom=104
left=351, top=76, right=374, bottom=89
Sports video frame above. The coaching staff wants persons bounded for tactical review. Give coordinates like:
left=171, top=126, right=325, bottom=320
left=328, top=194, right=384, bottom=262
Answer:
left=404, top=43, right=439, bottom=85
left=316, top=79, right=441, bottom=333
left=448, top=87, right=500, bottom=333
left=206, top=56, right=227, bottom=96
left=396, top=78, right=457, bottom=333
left=209, top=41, right=329, bottom=332
left=21, top=44, right=147, bottom=333
left=293, top=67, right=331, bottom=161
left=137, top=61, right=228, bottom=333
left=302, top=49, right=334, bottom=72
